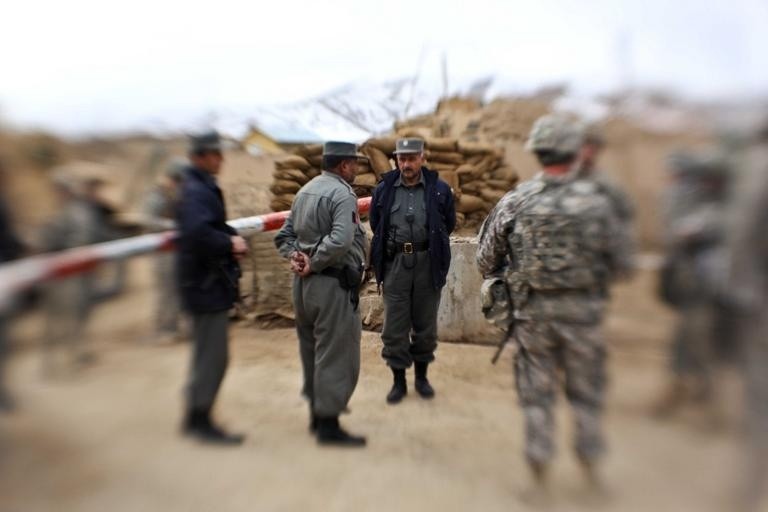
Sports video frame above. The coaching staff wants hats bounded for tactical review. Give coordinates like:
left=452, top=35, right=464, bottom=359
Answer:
left=183, top=128, right=233, bottom=152
left=322, top=141, right=370, bottom=158
left=392, top=138, right=426, bottom=154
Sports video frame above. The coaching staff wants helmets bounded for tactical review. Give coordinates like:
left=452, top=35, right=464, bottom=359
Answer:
left=526, top=115, right=584, bottom=156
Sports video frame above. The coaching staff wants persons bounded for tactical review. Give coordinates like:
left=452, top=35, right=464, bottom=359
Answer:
left=368, top=138, right=454, bottom=403
left=477, top=115, right=642, bottom=511
left=273, top=141, right=369, bottom=446
left=41, top=163, right=140, bottom=346
left=157, top=130, right=249, bottom=442
left=149, top=158, right=193, bottom=339
left=653, top=119, right=768, bottom=435
left=0, top=152, right=44, bottom=408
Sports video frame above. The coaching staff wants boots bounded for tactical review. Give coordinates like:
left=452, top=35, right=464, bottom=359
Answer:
left=318, top=415, right=366, bottom=447
left=414, top=359, right=434, bottom=397
left=386, top=366, right=407, bottom=402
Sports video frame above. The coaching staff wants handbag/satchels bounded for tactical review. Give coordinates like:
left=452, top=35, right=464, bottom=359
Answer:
left=478, top=275, right=512, bottom=342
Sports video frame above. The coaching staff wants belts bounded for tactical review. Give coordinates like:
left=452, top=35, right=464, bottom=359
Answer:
left=392, top=241, right=430, bottom=254
